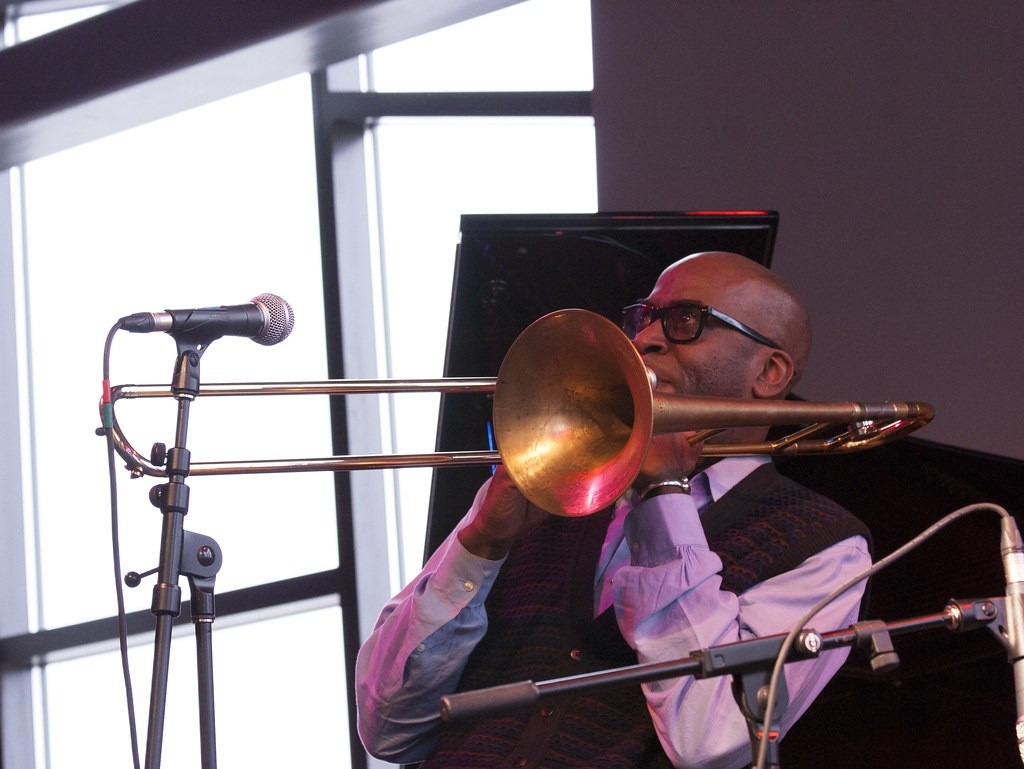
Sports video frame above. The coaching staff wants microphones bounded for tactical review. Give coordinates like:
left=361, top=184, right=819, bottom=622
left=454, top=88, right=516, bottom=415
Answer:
left=115, top=294, right=295, bottom=346
left=999, top=516, right=1024, bottom=765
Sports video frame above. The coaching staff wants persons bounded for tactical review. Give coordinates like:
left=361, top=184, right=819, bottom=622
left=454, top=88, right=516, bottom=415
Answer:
left=356, top=250, right=872, bottom=769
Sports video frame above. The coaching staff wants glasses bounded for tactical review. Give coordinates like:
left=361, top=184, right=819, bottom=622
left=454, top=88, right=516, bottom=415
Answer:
left=622, top=300, right=797, bottom=377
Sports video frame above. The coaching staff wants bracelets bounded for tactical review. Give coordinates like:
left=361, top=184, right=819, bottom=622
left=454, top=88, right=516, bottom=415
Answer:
left=639, top=477, right=690, bottom=501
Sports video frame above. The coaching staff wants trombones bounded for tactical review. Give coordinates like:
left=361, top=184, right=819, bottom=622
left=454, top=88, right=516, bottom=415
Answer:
left=98, top=308, right=932, bottom=518
